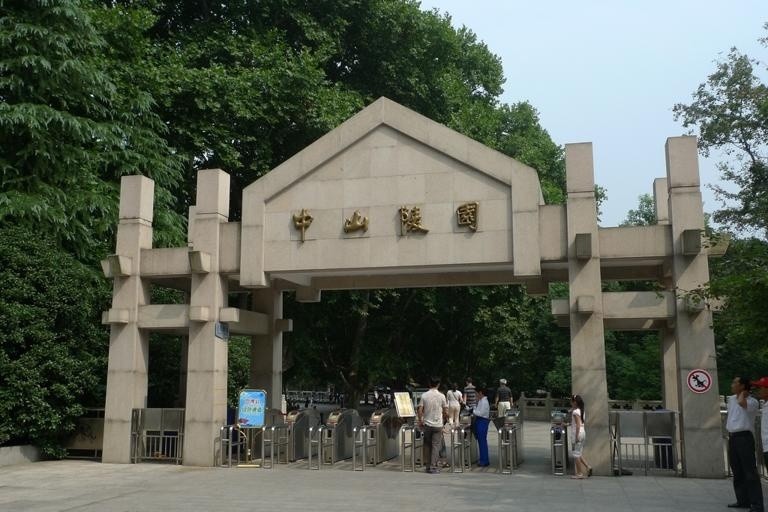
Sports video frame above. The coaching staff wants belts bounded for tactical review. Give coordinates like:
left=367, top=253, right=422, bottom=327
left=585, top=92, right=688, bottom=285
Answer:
left=729, top=431, right=751, bottom=437
left=499, top=400, right=509, bottom=401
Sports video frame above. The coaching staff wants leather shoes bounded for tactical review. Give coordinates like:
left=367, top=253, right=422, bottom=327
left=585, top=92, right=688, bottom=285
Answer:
left=728, top=502, right=764, bottom=512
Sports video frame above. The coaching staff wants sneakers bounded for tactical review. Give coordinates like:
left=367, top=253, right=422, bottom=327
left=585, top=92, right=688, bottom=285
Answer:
left=571, top=465, right=592, bottom=479
left=477, top=463, right=490, bottom=467
left=426, top=468, right=440, bottom=474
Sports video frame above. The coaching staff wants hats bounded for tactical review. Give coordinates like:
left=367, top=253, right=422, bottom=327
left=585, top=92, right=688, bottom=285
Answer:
left=499, top=379, right=506, bottom=384
left=751, top=377, right=768, bottom=387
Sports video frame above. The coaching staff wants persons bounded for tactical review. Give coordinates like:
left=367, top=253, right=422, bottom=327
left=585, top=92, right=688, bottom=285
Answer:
left=470, top=383, right=491, bottom=464
left=751, top=377, right=768, bottom=470
left=721, top=374, right=764, bottom=512
left=495, top=378, right=514, bottom=423
left=416, top=375, right=477, bottom=473
left=569, top=394, right=592, bottom=480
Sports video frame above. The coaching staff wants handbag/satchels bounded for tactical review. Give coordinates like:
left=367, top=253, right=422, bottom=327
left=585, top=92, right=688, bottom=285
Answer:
left=461, top=404, right=465, bottom=410
left=472, top=416, right=477, bottom=440
